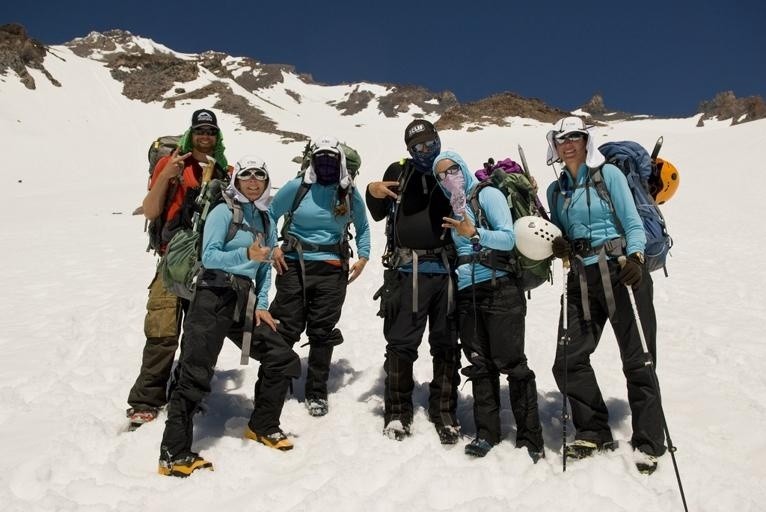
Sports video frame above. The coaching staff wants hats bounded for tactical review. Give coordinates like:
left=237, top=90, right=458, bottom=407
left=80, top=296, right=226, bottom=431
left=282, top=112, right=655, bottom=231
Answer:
left=312, top=138, right=341, bottom=154
left=404, top=119, right=438, bottom=150
left=191, top=108, right=220, bottom=130
left=554, top=116, right=589, bottom=138
left=234, top=156, right=268, bottom=175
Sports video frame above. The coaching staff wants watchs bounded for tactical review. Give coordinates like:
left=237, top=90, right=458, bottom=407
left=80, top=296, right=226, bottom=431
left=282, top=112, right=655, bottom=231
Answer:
left=469, top=230, right=480, bottom=245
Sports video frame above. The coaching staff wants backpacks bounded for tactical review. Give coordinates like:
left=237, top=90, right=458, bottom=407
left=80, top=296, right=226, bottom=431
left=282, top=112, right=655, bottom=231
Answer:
left=483, top=159, right=553, bottom=291
left=147, top=136, right=184, bottom=176
left=163, top=226, right=205, bottom=299
left=599, top=140, right=671, bottom=271
left=302, top=138, right=361, bottom=179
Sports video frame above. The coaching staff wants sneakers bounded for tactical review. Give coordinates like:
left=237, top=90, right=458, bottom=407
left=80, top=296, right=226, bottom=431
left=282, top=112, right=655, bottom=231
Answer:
left=465, top=437, right=500, bottom=457
left=129, top=411, right=156, bottom=428
left=527, top=448, right=545, bottom=464
left=158, top=452, right=213, bottom=478
left=632, top=444, right=659, bottom=475
left=305, top=397, right=329, bottom=416
left=560, top=438, right=615, bottom=460
left=438, top=424, right=460, bottom=445
left=384, top=424, right=411, bottom=440
left=244, top=423, right=295, bottom=451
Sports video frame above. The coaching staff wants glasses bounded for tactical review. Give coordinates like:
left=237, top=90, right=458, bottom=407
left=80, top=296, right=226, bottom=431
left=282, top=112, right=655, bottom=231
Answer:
left=412, top=140, right=435, bottom=152
left=194, top=128, right=217, bottom=136
left=556, top=133, right=581, bottom=143
left=238, top=170, right=267, bottom=180
left=436, top=164, right=458, bottom=182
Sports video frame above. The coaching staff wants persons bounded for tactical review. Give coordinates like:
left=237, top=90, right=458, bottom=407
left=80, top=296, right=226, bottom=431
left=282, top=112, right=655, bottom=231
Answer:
left=364, top=119, right=465, bottom=445
left=124, top=108, right=236, bottom=432
left=155, top=153, right=292, bottom=478
left=266, top=139, right=371, bottom=416
left=545, top=117, right=667, bottom=476
left=431, top=148, right=542, bottom=462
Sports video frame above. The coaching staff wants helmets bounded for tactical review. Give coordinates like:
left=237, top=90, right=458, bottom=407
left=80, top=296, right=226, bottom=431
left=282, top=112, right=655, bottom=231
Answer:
left=649, top=157, right=681, bottom=204
left=513, top=215, right=564, bottom=261
